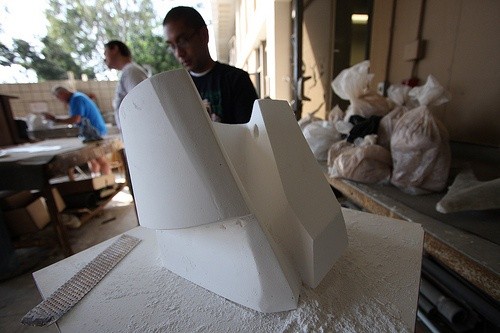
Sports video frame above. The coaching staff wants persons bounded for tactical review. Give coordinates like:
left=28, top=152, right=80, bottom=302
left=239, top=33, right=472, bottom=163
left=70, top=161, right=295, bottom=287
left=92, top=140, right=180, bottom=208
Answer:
left=43, top=86, right=110, bottom=176
left=103, top=40, right=148, bottom=226
left=162, top=6, right=260, bottom=124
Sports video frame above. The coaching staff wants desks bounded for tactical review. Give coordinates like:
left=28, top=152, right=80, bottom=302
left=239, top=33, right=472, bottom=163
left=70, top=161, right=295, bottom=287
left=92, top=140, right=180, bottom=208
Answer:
left=0, top=135, right=140, bottom=258
left=33, top=208, right=424, bottom=333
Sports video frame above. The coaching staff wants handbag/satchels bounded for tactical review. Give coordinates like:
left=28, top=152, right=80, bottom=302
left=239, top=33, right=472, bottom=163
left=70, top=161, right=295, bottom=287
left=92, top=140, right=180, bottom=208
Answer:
left=300, top=59, right=453, bottom=194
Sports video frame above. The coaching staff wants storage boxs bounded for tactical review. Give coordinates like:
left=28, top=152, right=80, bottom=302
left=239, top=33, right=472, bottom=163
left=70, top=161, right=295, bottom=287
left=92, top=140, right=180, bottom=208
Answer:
left=0, top=191, right=48, bottom=235
